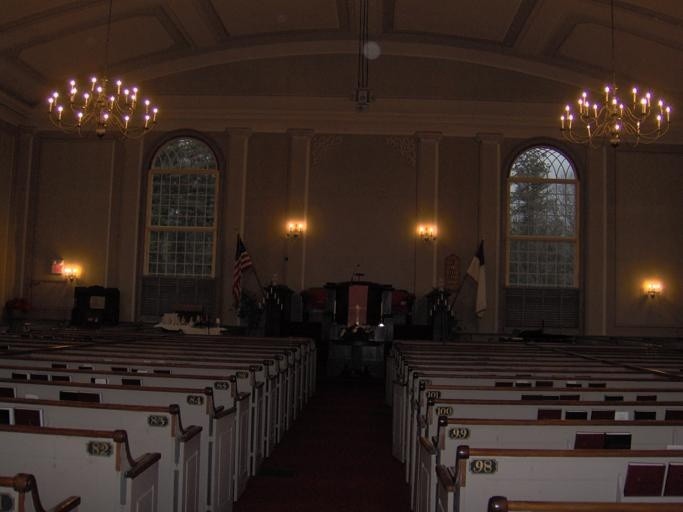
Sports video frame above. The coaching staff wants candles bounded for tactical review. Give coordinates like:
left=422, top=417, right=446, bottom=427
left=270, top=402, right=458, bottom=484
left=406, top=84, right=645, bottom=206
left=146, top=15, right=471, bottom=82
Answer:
left=432, top=292, right=452, bottom=312
left=258, top=285, right=284, bottom=310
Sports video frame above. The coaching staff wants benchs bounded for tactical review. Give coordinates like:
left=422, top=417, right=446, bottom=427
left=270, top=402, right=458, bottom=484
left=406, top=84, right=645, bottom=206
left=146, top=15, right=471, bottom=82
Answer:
left=2, top=326, right=320, bottom=511
left=381, top=336, right=683, bottom=510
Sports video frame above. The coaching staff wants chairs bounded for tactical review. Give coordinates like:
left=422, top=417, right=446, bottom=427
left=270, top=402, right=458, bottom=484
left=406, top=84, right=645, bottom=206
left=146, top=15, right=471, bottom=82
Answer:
left=299, top=285, right=336, bottom=320
left=381, top=289, right=416, bottom=324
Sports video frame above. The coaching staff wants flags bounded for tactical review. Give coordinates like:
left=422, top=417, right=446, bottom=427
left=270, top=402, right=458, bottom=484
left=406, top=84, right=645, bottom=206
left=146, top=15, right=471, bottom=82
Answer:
left=466, top=239, right=488, bottom=318
left=231, top=232, right=253, bottom=305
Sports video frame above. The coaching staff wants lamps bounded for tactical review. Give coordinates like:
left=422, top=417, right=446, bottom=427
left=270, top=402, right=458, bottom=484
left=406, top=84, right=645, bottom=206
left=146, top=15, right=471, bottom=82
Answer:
left=288, top=221, right=305, bottom=241
left=643, top=279, right=664, bottom=300
left=419, top=225, right=437, bottom=243
left=61, top=265, right=82, bottom=283
left=551, top=1, right=676, bottom=152
left=42, top=1, right=159, bottom=149
